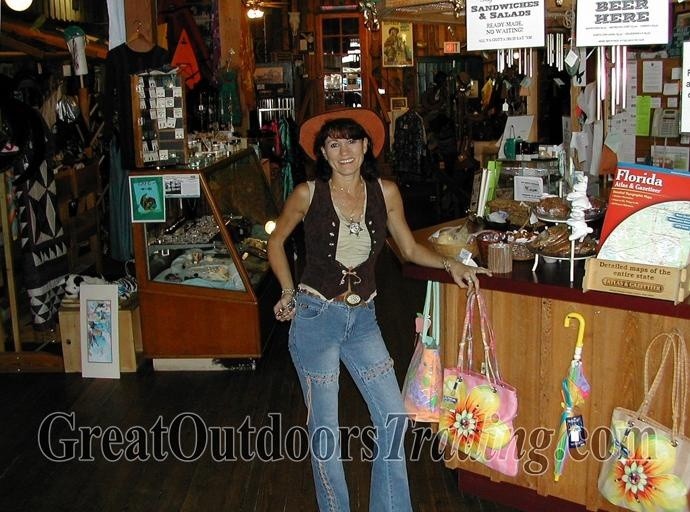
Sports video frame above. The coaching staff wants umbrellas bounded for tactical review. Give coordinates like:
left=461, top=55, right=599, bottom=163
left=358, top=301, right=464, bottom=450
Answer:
left=552, top=311, right=593, bottom=483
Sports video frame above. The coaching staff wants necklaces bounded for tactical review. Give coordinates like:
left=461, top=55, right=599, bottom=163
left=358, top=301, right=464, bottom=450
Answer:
left=328, top=180, right=364, bottom=238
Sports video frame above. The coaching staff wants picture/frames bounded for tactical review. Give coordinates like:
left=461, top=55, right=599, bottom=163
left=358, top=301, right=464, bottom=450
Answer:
left=380, top=20, right=415, bottom=67
left=79, top=281, right=123, bottom=381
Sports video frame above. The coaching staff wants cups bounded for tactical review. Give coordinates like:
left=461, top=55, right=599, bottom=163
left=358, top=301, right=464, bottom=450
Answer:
left=486, top=242, right=513, bottom=273
left=503, top=138, right=515, bottom=160
left=476, top=230, right=501, bottom=262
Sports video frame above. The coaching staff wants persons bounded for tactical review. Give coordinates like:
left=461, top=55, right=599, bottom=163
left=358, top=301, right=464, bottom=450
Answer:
left=384, top=28, right=401, bottom=63
left=265, top=102, right=496, bottom=511
left=454, top=65, right=474, bottom=96
left=423, top=71, right=450, bottom=108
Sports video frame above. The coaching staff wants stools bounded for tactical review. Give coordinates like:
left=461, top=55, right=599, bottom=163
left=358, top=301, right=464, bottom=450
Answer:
left=59, top=282, right=143, bottom=379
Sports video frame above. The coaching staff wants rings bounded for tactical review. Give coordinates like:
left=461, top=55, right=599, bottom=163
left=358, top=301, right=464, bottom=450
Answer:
left=467, top=276, right=473, bottom=283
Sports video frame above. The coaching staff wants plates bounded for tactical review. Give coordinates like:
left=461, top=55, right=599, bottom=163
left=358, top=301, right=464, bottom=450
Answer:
left=537, top=214, right=604, bottom=224
left=539, top=252, right=597, bottom=262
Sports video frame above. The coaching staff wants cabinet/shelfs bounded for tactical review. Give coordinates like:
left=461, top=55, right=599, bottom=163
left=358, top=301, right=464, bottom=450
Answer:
left=130, top=143, right=291, bottom=359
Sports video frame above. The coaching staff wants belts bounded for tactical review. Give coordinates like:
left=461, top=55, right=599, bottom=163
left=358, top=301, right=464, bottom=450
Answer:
left=296, top=287, right=364, bottom=307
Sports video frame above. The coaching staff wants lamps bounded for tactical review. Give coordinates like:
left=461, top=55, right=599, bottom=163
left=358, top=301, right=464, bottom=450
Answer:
left=246, top=0, right=264, bottom=23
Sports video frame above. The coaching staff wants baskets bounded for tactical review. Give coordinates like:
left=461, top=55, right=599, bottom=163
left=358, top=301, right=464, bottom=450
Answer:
left=427, top=232, right=480, bottom=261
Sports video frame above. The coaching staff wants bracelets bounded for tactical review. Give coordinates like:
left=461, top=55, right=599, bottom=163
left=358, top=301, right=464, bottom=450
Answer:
left=441, top=255, right=457, bottom=274
left=280, top=288, right=296, bottom=297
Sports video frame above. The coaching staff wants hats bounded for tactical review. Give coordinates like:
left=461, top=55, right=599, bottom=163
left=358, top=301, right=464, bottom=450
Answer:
left=298, top=104, right=385, bottom=162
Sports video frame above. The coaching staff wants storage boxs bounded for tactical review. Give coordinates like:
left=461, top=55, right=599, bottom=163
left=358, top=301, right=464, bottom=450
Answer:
left=582, top=255, right=690, bottom=307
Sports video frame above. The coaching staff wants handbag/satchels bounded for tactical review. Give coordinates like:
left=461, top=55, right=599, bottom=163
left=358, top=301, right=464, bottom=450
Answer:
left=597, top=406, right=690, bottom=512
left=401, top=334, right=444, bottom=423
left=437, top=367, right=519, bottom=477
